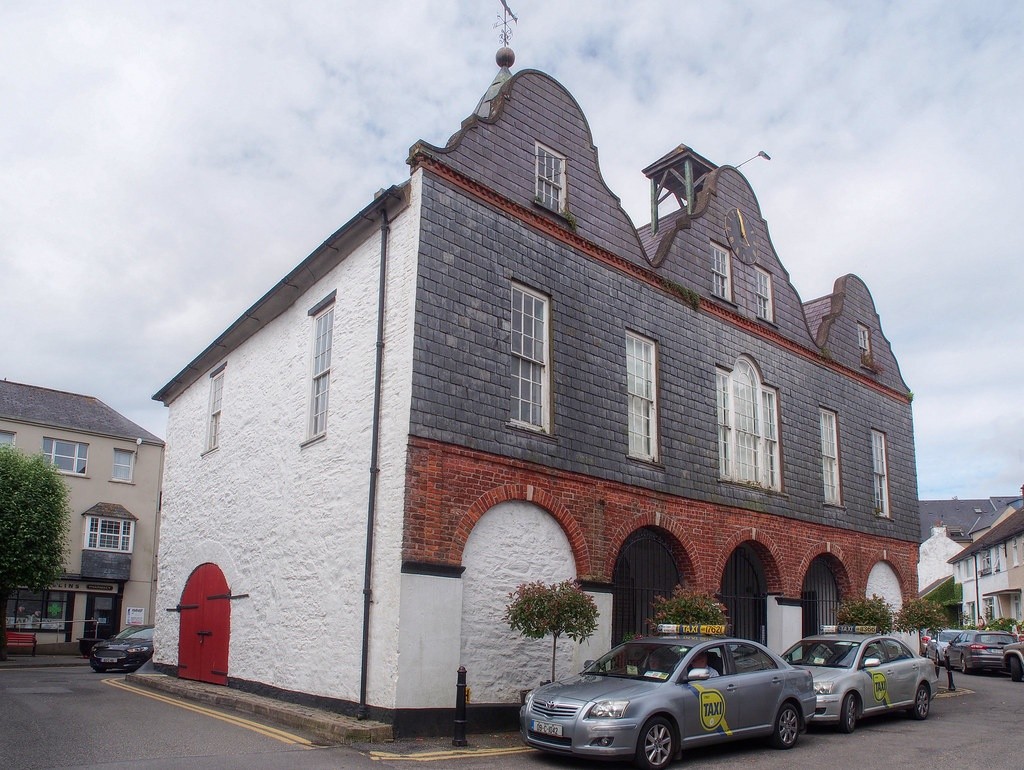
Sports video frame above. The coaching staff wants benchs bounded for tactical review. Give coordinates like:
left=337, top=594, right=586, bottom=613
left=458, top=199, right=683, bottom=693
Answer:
left=6, top=631, right=37, bottom=656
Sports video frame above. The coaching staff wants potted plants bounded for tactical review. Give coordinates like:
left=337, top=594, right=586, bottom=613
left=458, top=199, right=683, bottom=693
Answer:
left=500, top=578, right=600, bottom=706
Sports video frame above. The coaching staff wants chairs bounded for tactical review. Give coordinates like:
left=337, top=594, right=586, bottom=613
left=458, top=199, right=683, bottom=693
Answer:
left=644, top=647, right=677, bottom=675
left=688, top=652, right=722, bottom=681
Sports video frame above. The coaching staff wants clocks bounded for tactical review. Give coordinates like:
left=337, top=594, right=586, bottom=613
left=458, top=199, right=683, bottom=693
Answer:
left=724, top=208, right=759, bottom=265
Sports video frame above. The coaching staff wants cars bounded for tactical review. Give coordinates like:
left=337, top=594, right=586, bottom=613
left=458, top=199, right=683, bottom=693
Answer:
left=771, top=623, right=938, bottom=732
left=925, top=630, right=966, bottom=666
left=519, top=625, right=817, bottom=770
left=90, top=625, right=155, bottom=674
left=920, top=626, right=938, bottom=657
left=943, top=629, right=1018, bottom=675
left=1002, top=641, right=1024, bottom=682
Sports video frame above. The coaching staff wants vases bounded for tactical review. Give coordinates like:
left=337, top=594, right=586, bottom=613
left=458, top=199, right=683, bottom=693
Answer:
left=76, top=637, right=105, bottom=658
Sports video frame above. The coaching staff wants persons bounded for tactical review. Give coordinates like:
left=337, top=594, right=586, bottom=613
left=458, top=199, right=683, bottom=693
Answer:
left=640, top=651, right=666, bottom=677
left=688, top=651, right=721, bottom=678
